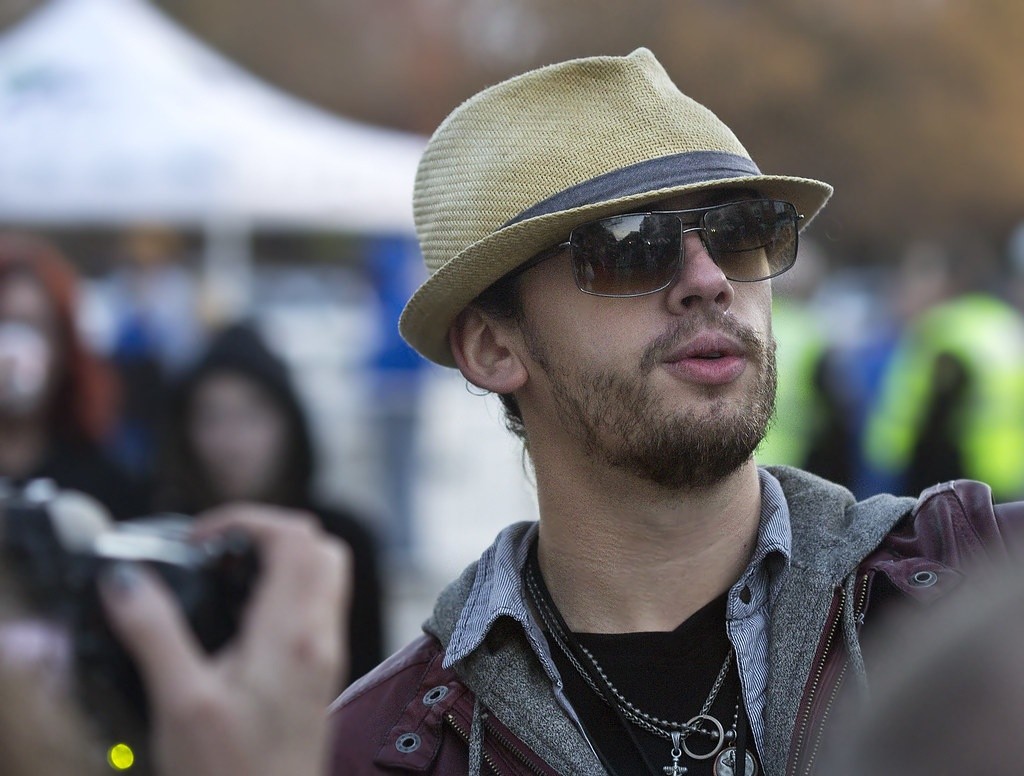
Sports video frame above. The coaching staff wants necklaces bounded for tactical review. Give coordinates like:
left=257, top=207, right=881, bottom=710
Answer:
left=518, top=557, right=749, bottom=776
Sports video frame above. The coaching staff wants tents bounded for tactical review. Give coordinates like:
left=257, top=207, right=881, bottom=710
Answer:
left=1, top=0, right=432, bottom=311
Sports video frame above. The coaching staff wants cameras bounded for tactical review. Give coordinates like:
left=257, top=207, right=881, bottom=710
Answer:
left=0, top=474, right=259, bottom=743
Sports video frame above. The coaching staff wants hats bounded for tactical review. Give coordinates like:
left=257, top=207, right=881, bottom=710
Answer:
left=398, top=46, right=834, bottom=368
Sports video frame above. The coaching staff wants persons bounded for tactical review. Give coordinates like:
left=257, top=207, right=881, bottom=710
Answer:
left=322, top=45, right=1024, bottom=776
left=1, top=228, right=385, bottom=776
left=751, top=234, right=1024, bottom=504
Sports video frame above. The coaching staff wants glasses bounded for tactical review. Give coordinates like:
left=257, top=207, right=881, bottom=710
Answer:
left=509, top=198, right=804, bottom=298
